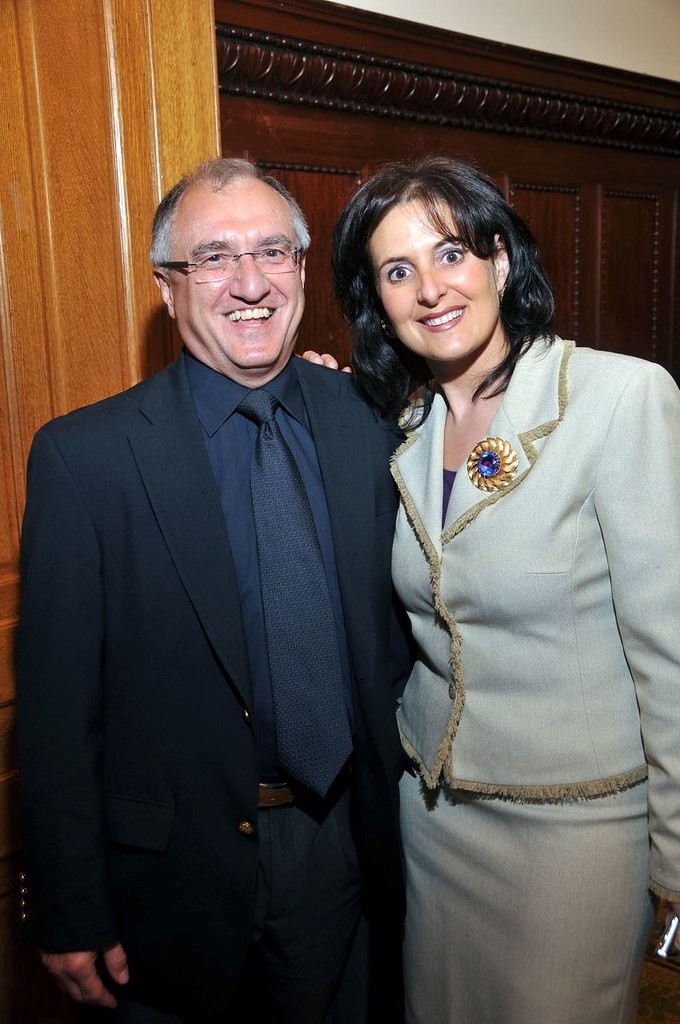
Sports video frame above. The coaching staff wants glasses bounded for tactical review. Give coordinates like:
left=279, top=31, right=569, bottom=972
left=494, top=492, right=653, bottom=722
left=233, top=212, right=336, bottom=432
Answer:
left=157, top=244, right=303, bottom=283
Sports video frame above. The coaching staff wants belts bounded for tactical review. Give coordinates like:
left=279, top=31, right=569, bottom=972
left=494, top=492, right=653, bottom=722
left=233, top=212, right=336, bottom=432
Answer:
left=259, top=788, right=294, bottom=806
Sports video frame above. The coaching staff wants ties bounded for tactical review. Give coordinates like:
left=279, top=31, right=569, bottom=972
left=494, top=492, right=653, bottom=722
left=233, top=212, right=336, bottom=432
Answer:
left=235, top=390, right=353, bottom=796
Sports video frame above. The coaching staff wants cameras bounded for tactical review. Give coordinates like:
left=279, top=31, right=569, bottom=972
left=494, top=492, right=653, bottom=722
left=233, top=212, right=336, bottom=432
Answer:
left=656, top=917, right=678, bottom=958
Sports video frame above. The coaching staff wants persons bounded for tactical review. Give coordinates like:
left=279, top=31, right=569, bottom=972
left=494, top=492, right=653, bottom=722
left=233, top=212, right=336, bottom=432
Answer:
left=0, top=157, right=417, bottom=1023
left=287, top=156, right=679, bottom=1023
